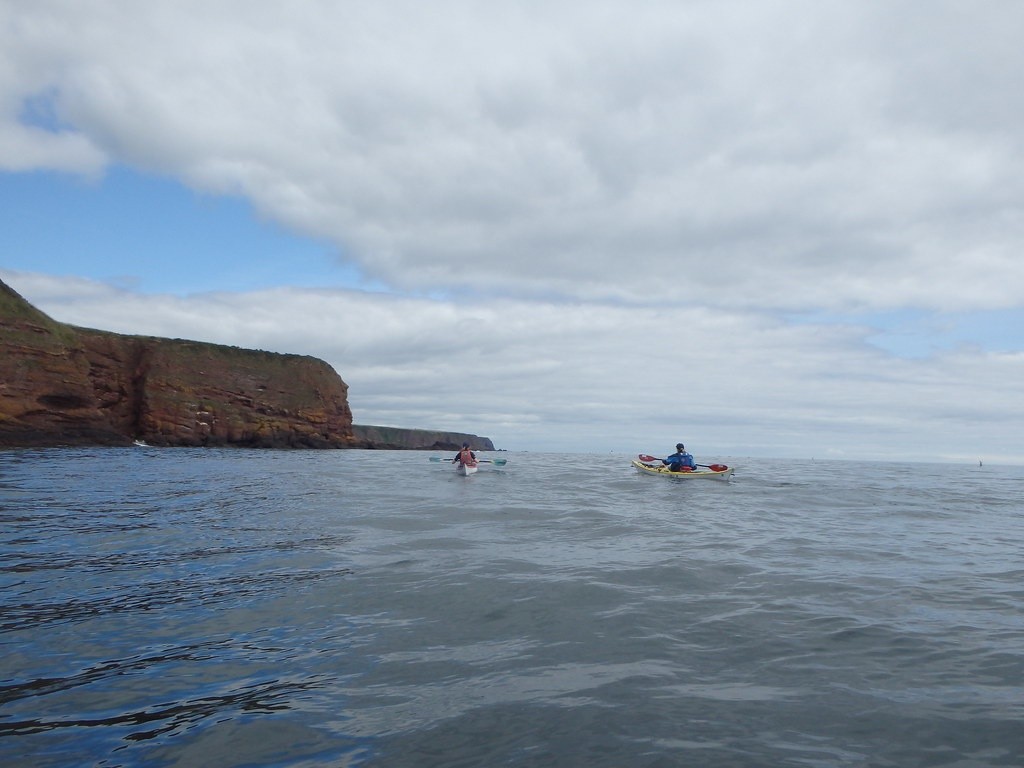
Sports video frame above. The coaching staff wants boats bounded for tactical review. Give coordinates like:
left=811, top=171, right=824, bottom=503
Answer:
left=631, top=459, right=736, bottom=481
left=456, top=462, right=478, bottom=476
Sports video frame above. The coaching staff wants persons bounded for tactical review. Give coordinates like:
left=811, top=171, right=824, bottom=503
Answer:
left=452, top=441, right=480, bottom=464
left=662, top=443, right=697, bottom=472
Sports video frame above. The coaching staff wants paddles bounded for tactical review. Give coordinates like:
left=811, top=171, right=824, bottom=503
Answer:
left=429, top=457, right=507, bottom=466
left=639, top=454, right=728, bottom=472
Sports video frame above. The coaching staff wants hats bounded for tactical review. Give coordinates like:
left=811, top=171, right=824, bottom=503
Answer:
left=463, top=441, right=470, bottom=448
left=676, top=443, right=684, bottom=450
